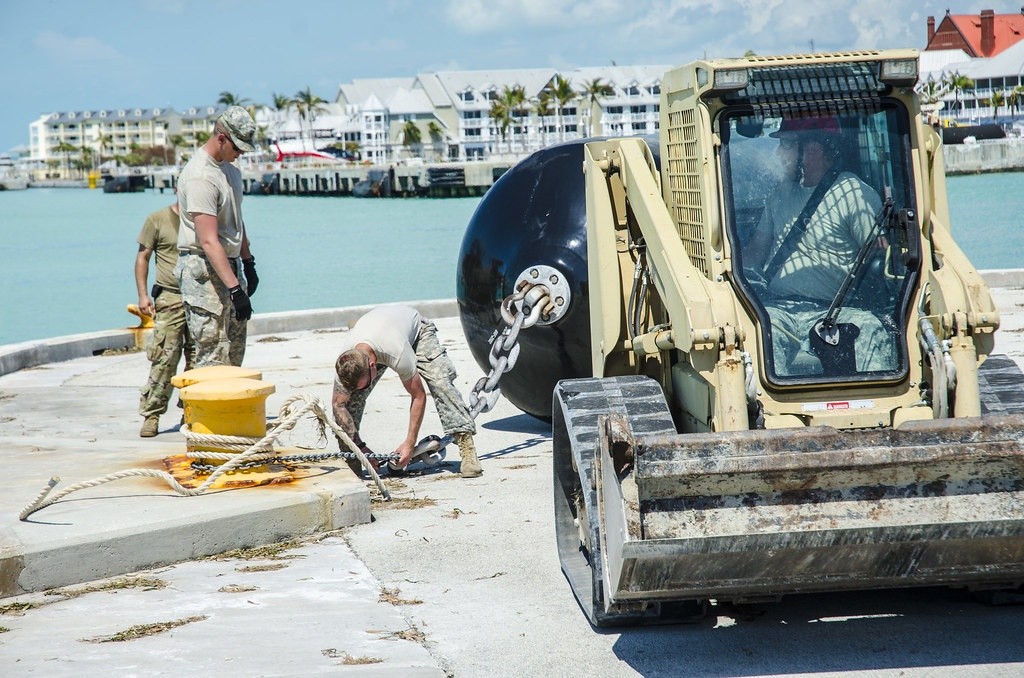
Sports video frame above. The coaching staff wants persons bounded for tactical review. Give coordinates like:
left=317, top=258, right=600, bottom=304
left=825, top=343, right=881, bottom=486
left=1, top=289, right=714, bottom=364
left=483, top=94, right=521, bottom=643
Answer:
left=135, top=202, right=196, bottom=436
left=740, top=118, right=898, bottom=376
left=331, top=302, right=482, bottom=478
left=174, top=105, right=259, bottom=367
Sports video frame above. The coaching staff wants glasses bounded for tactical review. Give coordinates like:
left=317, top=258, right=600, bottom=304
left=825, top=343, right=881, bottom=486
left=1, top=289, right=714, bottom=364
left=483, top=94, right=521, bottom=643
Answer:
left=218, top=129, right=241, bottom=152
left=356, top=359, right=372, bottom=391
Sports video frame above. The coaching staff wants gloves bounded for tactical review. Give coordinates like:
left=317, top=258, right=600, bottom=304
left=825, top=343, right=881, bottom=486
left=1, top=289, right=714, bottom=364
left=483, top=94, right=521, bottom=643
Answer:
left=229, top=285, right=252, bottom=322
left=243, top=258, right=259, bottom=297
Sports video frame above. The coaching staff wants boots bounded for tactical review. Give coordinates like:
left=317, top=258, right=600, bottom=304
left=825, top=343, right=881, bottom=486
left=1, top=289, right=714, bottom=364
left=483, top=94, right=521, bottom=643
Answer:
left=453, top=431, right=481, bottom=477
left=338, top=438, right=361, bottom=474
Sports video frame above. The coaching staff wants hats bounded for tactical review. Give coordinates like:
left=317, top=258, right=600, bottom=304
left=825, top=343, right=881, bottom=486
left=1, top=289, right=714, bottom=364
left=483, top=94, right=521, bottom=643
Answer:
left=219, top=106, right=257, bottom=152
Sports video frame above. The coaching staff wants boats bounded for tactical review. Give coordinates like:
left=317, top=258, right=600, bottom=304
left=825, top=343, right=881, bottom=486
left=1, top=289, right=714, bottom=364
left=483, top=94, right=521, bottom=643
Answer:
left=1, top=152, right=31, bottom=190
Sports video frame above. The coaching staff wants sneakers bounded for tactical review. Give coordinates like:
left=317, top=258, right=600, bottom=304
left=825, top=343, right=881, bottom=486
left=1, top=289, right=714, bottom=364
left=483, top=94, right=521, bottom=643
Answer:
left=140, top=416, right=158, bottom=437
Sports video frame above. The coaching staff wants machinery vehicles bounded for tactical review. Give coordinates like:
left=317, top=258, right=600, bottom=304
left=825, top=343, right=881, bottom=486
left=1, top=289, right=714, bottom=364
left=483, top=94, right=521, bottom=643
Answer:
left=552, top=47, right=1024, bottom=629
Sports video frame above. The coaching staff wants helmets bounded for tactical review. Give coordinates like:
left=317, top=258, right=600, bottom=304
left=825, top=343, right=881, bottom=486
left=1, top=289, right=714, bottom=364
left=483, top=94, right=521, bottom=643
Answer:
left=769, top=105, right=840, bottom=140
